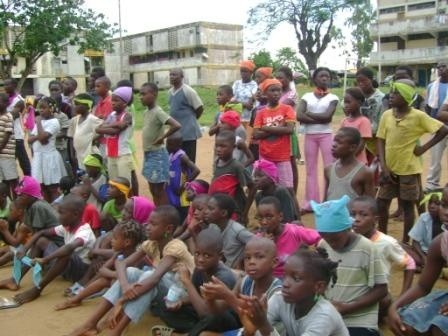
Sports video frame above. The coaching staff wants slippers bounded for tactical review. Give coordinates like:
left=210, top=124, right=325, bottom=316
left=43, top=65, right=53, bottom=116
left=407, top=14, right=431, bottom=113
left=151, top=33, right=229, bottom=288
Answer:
left=64, top=282, right=102, bottom=300
left=151, top=325, right=176, bottom=336
left=0, top=295, right=18, bottom=309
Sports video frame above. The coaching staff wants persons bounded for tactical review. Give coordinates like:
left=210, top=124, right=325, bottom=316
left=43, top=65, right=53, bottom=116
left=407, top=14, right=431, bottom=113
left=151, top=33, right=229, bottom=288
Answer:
left=94, top=86, right=140, bottom=197
left=0, top=67, right=155, bottom=311
left=220, top=110, right=254, bottom=168
left=138, top=81, right=181, bottom=208
left=253, top=79, right=295, bottom=201
left=247, top=185, right=321, bottom=282
left=64, top=205, right=194, bottom=336
left=347, top=195, right=416, bottom=321
left=208, top=130, right=256, bottom=226
left=168, top=68, right=204, bottom=166
left=321, top=58, right=448, bottom=336
left=153, top=173, right=260, bottom=273
left=151, top=229, right=240, bottom=336
left=304, top=194, right=388, bottom=336
left=242, top=244, right=349, bottom=336
left=209, top=60, right=299, bottom=197
left=296, top=67, right=339, bottom=217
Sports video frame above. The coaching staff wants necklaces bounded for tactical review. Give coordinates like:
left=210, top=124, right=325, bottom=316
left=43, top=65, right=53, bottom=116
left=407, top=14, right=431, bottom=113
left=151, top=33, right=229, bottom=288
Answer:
left=391, top=113, right=407, bottom=122
